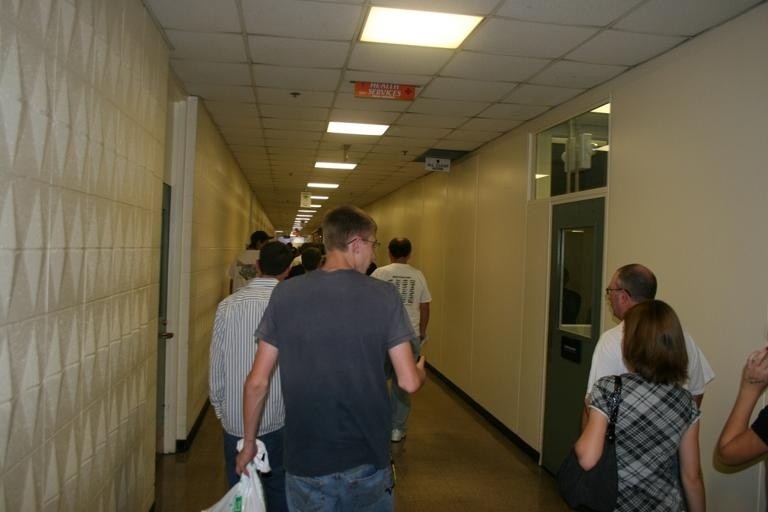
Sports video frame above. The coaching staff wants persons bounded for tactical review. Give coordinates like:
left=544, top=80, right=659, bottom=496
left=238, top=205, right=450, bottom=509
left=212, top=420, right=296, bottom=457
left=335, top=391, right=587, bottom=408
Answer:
left=575, top=299, right=706, bottom=512
left=229, top=230, right=377, bottom=295
left=581, top=264, right=716, bottom=442
left=369, top=238, right=432, bottom=442
left=235, top=204, right=427, bottom=512
left=207, top=240, right=294, bottom=512
left=712, top=344, right=768, bottom=475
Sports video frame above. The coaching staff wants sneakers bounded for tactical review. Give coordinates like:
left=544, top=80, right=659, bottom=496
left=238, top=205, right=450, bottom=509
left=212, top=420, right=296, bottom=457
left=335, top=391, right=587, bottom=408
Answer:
left=392, top=426, right=408, bottom=442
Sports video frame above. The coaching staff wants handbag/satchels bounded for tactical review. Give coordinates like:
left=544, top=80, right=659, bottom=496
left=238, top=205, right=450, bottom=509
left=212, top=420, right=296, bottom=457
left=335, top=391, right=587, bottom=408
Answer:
left=553, top=428, right=620, bottom=512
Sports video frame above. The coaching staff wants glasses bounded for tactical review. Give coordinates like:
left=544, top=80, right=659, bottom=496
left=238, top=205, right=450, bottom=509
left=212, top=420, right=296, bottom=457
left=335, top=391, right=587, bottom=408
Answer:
left=347, top=236, right=381, bottom=253
left=605, top=287, right=632, bottom=297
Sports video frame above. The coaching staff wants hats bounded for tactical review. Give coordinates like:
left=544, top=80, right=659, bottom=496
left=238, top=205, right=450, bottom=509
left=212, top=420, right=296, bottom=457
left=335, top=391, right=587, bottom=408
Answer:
left=250, top=230, right=275, bottom=244
